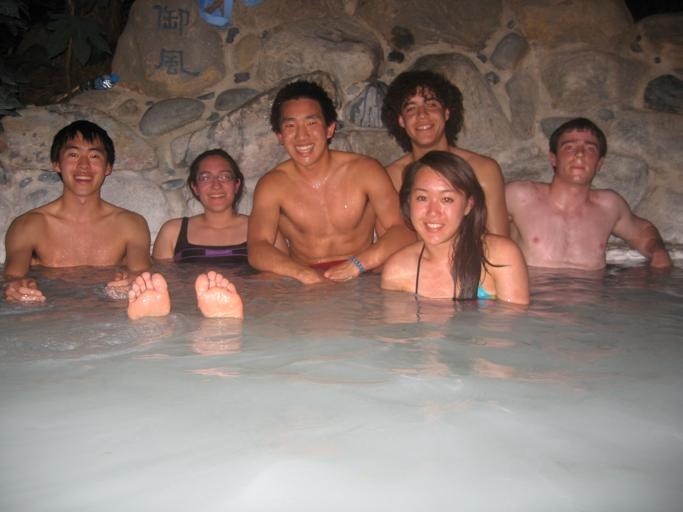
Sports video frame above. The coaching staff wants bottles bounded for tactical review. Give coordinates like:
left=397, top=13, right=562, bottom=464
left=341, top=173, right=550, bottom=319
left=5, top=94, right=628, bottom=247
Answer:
left=81, top=72, right=120, bottom=90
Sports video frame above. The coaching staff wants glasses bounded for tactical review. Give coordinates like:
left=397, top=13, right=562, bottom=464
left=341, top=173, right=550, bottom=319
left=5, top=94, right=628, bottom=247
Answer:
left=201, top=172, right=233, bottom=183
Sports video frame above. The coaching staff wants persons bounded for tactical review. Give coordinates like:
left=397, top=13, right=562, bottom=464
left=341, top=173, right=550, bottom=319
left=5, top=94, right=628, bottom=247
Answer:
left=502, top=119, right=672, bottom=272
left=374, top=71, right=510, bottom=237
left=379, top=150, right=530, bottom=304
left=1, top=120, right=151, bottom=303
left=126, top=149, right=290, bottom=319
left=247, top=81, right=419, bottom=286
